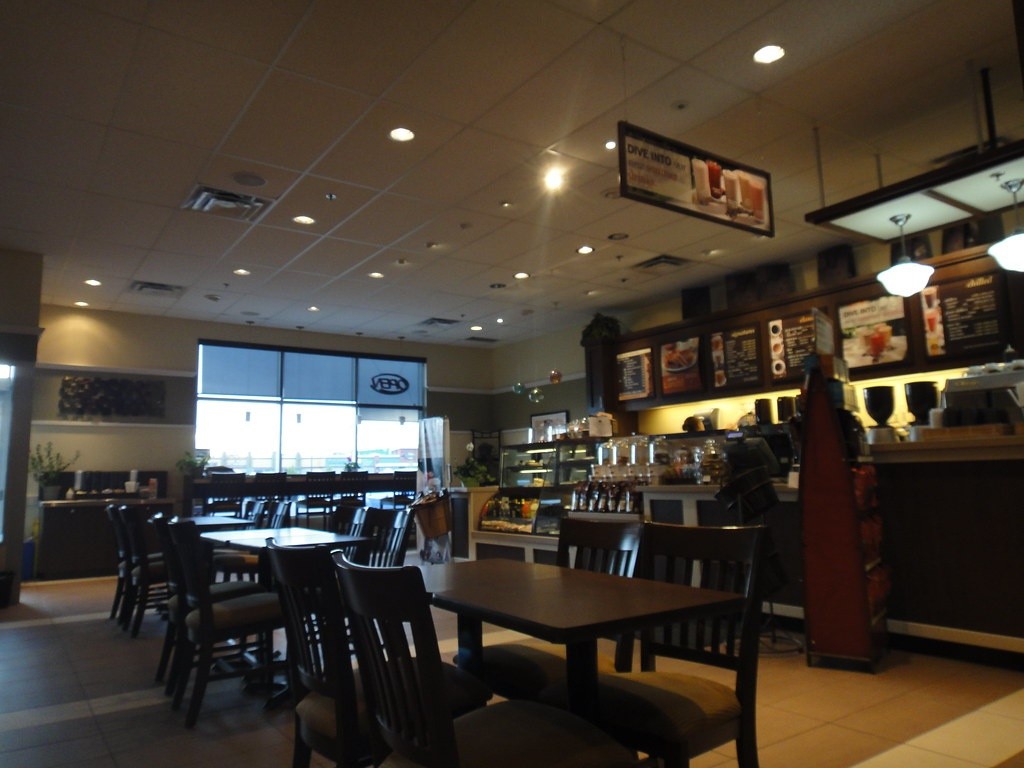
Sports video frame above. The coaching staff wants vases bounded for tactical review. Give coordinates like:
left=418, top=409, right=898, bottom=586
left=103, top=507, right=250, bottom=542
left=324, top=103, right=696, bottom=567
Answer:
left=348, top=467, right=357, bottom=473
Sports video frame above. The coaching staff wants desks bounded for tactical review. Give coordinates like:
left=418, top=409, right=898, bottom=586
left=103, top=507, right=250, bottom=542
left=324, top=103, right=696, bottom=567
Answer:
left=421, top=557, right=747, bottom=725
left=199, top=527, right=378, bottom=708
left=183, top=515, right=254, bottom=534
left=181, top=474, right=409, bottom=518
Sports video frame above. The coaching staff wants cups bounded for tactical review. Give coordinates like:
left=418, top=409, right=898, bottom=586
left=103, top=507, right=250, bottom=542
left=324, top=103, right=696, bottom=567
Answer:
left=692, top=158, right=764, bottom=224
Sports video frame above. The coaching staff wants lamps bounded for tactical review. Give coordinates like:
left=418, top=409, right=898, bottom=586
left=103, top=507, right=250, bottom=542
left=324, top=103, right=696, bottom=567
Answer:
left=875, top=213, right=935, bottom=297
left=989, top=179, right=1024, bottom=275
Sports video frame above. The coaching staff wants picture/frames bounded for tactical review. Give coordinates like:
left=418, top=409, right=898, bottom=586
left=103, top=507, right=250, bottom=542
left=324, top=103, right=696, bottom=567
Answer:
left=530, top=410, right=568, bottom=429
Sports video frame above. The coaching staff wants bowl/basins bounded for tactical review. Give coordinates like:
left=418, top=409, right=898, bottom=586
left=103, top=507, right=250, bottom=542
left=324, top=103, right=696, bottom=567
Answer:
left=73, top=492, right=136, bottom=499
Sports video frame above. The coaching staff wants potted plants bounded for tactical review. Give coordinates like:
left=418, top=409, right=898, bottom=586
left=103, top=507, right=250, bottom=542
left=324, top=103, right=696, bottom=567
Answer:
left=25, top=441, right=80, bottom=499
left=449, top=442, right=497, bottom=487
left=177, top=451, right=211, bottom=479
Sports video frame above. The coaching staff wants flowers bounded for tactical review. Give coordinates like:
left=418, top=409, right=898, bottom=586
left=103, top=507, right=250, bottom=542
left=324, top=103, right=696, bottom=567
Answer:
left=343, top=458, right=361, bottom=470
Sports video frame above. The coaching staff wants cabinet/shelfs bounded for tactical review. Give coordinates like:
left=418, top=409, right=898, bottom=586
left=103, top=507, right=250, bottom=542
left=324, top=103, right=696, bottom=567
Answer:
left=36, top=500, right=172, bottom=582
left=480, top=456, right=595, bottom=534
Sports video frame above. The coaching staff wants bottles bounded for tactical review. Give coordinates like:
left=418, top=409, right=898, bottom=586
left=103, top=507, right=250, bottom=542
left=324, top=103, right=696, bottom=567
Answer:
left=480, top=496, right=539, bottom=520
left=573, top=481, right=647, bottom=515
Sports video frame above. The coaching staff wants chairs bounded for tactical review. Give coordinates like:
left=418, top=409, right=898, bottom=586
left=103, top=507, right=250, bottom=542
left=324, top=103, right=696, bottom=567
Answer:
left=220, top=496, right=292, bottom=588
left=104, top=503, right=176, bottom=635
left=150, top=511, right=300, bottom=728
left=296, top=472, right=337, bottom=531
left=380, top=470, right=419, bottom=511
left=246, top=472, right=291, bottom=525
left=198, top=473, right=246, bottom=517
left=331, top=471, right=369, bottom=521
left=296, top=508, right=416, bottom=669
left=263, top=536, right=634, bottom=768
left=452, top=512, right=766, bottom=768
